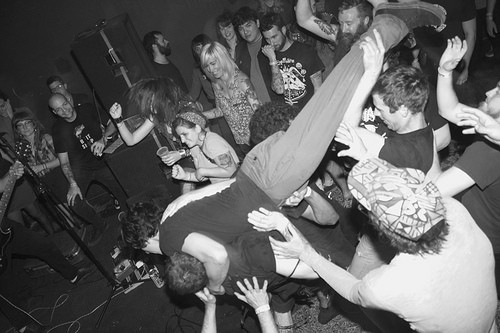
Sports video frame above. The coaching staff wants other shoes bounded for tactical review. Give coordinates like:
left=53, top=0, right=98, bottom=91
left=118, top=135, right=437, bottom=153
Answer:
left=375, top=1, right=446, bottom=35
left=318, top=306, right=339, bottom=325
left=343, top=197, right=353, bottom=209
left=322, top=183, right=337, bottom=194
left=68, top=263, right=98, bottom=287
left=485, top=47, right=495, bottom=58
left=88, top=220, right=110, bottom=249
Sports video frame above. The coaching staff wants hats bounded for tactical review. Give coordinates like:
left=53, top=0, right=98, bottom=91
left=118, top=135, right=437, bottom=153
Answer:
left=347, top=157, right=447, bottom=244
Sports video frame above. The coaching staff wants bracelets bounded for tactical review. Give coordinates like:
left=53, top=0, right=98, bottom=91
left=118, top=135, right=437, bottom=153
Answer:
left=179, top=149, right=186, bottom=158
left=486, top=14, right=494, bottom=20
left=41, top=163, right=45, bottom=171
left=69, top=183, right=78, bottom=187
left=255, top=304, right=270, bottom=314
left=269, top=61, right=278, bottom=65
left=114, top=116, right=124, bottom=123
left=187, top=172, right=191, bottom=180
left=118, top=121, right=124, bottom=128
left=43, top=162, right=47, bottom=169
left=438, top=68, right=453, bottom=77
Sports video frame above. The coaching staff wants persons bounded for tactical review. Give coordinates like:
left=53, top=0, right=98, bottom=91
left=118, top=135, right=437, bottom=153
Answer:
left=268, top=122, right=496, bottom=333
left=170, top=107, right=240, bottom=185
left=47, top=94, right=129, bottom=245
left=433, top=37, right=500, bottom=300
left=0, top=0, right=500, bottom=333
left=336, top=28, right=440, bottom=181
left=229, top=6, right=281, bottom=107
left=200, top=40, right=262, bottom=155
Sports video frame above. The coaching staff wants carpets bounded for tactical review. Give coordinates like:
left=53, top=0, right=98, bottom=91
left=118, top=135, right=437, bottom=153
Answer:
left=286, top=141, right=463, bottom=333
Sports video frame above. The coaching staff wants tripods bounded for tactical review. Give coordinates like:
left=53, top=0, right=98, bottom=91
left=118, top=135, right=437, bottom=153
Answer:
left=0, top=135, right=164, bottom=330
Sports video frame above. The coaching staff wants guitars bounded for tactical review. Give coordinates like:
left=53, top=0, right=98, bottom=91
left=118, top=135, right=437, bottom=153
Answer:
left=0, top=138, right=31, bottom=273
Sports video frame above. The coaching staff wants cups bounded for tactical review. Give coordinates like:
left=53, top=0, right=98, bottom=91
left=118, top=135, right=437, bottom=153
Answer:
left=156, top=146, right=169, bottom=157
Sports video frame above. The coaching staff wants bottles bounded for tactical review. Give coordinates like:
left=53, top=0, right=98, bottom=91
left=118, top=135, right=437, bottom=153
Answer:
left=148, top=268, right=165, bottom=289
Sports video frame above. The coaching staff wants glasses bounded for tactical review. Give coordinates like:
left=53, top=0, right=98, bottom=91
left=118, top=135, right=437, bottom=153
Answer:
left=51, top=83, right=64, bottom=93
left=16, top=121, right=32, bottom=129
